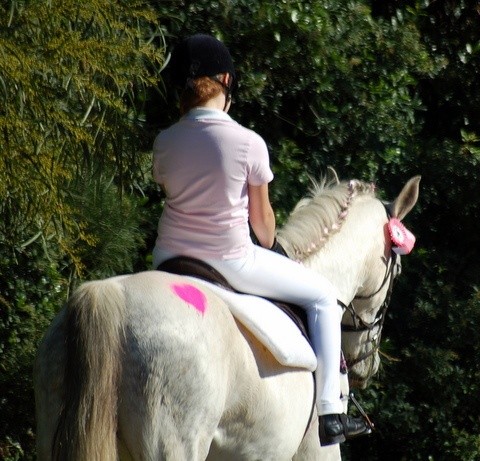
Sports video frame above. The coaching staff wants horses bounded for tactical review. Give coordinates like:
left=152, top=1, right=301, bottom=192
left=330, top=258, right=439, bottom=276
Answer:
left=34, top=166, right=422, bottom=461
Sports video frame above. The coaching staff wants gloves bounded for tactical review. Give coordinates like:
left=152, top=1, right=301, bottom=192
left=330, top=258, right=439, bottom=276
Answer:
left=256, top=237, right=289, bottom=258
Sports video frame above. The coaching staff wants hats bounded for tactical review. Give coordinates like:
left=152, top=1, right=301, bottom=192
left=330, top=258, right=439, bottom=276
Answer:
left=165, top=35, right=236, bottom=84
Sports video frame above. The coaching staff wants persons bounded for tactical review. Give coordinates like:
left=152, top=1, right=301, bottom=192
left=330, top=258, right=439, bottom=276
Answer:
left=149, top=35, right=367, bottom=446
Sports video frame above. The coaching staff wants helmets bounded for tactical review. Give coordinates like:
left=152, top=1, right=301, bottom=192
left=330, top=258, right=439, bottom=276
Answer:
left=319, top=413, right=367, bottom=446
